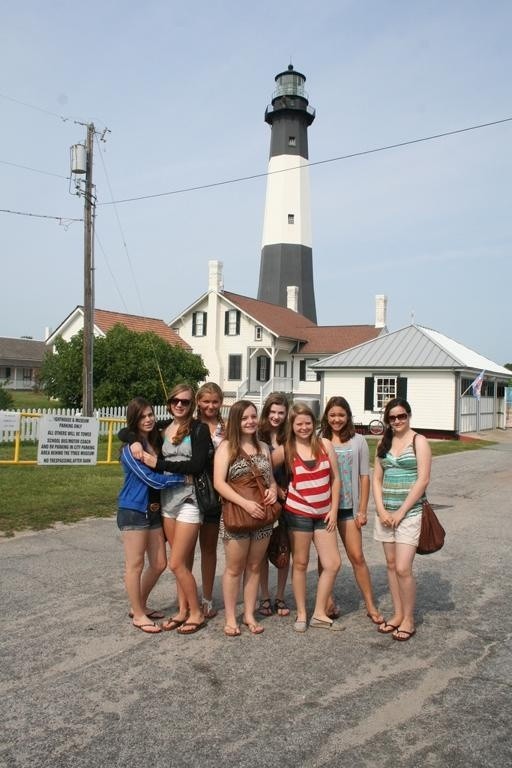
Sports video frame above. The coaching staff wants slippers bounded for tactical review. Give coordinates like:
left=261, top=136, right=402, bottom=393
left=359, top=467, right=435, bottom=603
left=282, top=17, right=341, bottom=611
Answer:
left=225, top=599, right=289, bottom=636
left=368, top=612, right=415, bottom=641
left=129, top=596, right=216, bottom=634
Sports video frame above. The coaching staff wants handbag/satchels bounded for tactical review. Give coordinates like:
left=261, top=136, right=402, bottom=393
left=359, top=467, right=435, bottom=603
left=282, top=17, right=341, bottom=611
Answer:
left=221, top=448, right=282, bottom=531
left=268, top=522, right=289, bottom=569
left=194, top=424, right=218, bottom=515
left=416, top=502, right=445, bottom=554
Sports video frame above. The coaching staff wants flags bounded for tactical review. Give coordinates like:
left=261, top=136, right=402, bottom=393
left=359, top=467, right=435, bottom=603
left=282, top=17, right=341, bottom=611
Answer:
left=471, top=371, right=484, bottom=401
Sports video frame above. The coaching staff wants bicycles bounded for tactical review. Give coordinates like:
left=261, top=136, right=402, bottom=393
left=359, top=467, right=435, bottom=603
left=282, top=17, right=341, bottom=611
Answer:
left=367, top=403, right=389, bottom=436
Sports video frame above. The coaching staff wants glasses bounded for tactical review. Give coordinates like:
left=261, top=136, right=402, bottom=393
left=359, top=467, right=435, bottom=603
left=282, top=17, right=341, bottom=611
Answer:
left=170, top=398, right=192, bottom=405
left=389, top=414, right=407, bottom=421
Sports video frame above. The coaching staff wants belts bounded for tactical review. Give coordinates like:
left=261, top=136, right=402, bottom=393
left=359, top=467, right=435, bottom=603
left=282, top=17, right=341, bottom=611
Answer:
left=147, top=503, right=161, bottom=512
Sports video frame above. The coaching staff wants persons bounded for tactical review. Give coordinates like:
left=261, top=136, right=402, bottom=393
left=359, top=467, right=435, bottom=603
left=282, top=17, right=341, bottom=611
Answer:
left=256, top=391, right=293, bottom=616
left=117, top=383, right=226, bottom=638
left=269, top=402, right=347, bottom=632
left=212, top=399, right=277, bottom=639
left=319, top=395, right=383, bottom=623
left=372, top=398, right=432, bottom=643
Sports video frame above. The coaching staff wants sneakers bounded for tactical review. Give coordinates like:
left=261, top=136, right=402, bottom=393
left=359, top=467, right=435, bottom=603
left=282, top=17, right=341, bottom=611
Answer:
left=309, top=616, right=345, bottom=631
left=293, top=616, right=308, bottom=632
